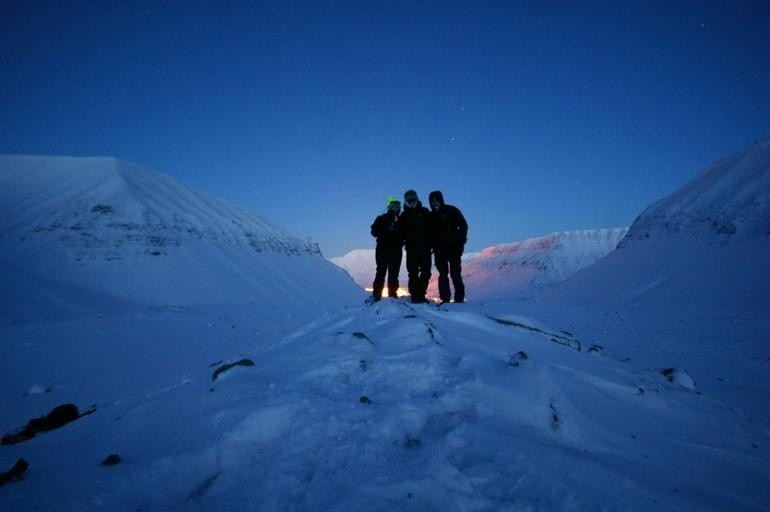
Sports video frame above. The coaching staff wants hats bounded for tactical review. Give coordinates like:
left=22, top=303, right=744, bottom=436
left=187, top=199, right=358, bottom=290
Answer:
left=387, top=197, right=402, bottom=211
left=404, top=189, right=418, bottom=202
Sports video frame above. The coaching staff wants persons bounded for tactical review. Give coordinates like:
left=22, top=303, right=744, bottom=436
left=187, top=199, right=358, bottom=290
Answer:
left=428, top=191, right=468, bottom=304
left=365, top=196, right=401, bottom=303
left=392, top=189, right=436, bottom=305
